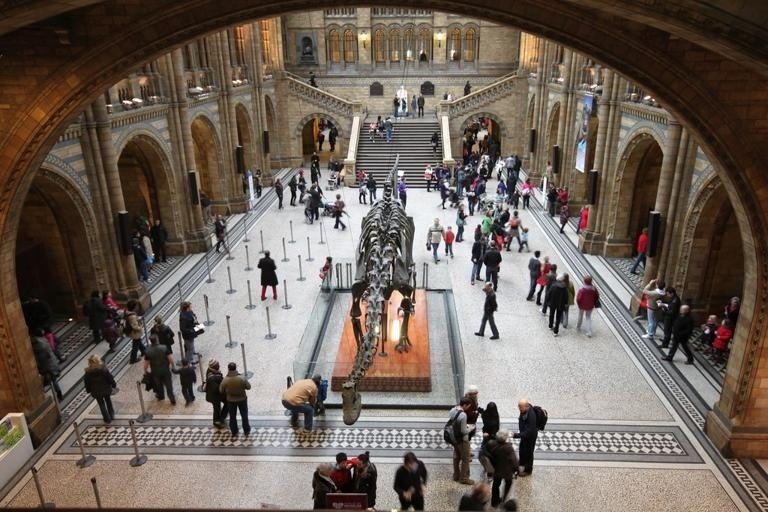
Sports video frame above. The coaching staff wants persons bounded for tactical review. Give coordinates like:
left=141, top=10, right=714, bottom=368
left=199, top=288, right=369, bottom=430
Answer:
left=21, top=286, right=252, bottom=439
left=527, top=250, right=599, bottom=339
left=398, top=177, right=408, bottom=209
left=475, top=282, right=499, bottom=339
left=214, top=214, right=230, bottom=253
left=308, top=71, right=318, bottom=88
left=242, top=126, right=377, bottom=231
left=281, top=376, right=319, bottom=433
left=444, top=91, right=448, bottom=100
left=463, top=120, right=498, bottom=178
left=447, top=92, right=452, bottom=101
left=457, top=200, right=529, bottom=291
left=198, top=189, right=213, bottom=227
left=311, top=387, right=539, bottom=512
left=318, top=256, right=333, bottom=281
left=427, top=217, right=455, bottom=263
left=312, top=375, right=328, bottom=417
left=480, top=152, right=532, bottom=209
left=366, top=91, right=425, bottom=143
left=631, top=227, right=648, bottom=275
left=641, top=276, right=740, bottom=364
left=547, top=183, right=589, bottom=233
left=130, top=213, right=168, bottom=284
left=430, top=133, right=439, bottom=153
left=425, top=164, right=486, bottom=216
left=546, top=160, right=559, bottom=186
left=257, top=250, right=278, bottom=300
left=463, top=81, right=472, bottom=96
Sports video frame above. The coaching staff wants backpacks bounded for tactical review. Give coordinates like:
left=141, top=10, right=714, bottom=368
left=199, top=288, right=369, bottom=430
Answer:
left=444, top=410, right=462, bottom=445
left=478, top=440, right=500, bottom=475
left=114, top=309, right=136, bottom=337
left=529, top=403, right=547, bottom=430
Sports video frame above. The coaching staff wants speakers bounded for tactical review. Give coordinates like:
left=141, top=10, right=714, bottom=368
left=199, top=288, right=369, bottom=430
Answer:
left=552, top=145, right=560, bottom=174
left=587, top=169, right=598, bottom=205
left=236, top=146, right=245, bottom=174
left=117, top=209, right=133, bottom=256
left=189, top=171, right=199, bottom=206
left=529, top=129, right=535, bottom=152
left=646, top=210, right=660, bottom=256
left=264, top=130, right=270, bottom=155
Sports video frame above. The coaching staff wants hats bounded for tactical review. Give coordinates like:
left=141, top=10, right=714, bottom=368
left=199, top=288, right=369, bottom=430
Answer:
left=208, top=358, right=218, bottom=368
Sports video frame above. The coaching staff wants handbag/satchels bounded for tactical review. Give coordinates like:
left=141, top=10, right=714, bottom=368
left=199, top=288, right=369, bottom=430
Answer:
left=106, top=385, right=119, bottom=395
left=594, top=297, right=601, bottom=309
left=426, top=242, right=431, bottom=250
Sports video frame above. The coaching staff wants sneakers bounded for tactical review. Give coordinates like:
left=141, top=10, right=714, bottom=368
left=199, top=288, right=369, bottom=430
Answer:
left=213, top=421, right=226, bottom=428
left=684, top=357, right=694, bottom=364
left=460, top=478, right=475, bottom=485
left=527, top=297, right=535, bottom=301
left=471, top=280, right=474, bottom=285
left=518, top=469, right=532, bottom=478
left=661, top=356, right=673, bottom=361
left=641, top=334, right=653, bottom=339
left=490, top=336, right=499, bottom=339
left=475, top=332, right=484, bottom=337
left=477, top=278, right=483, bottom=281
left=553, top=327, right=558, bottom=336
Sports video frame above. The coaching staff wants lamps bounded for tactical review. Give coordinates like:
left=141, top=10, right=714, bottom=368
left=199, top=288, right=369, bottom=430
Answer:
left=437, top=29, right=443, bottom=48
left=103, top=72, right=274, bottom=117
left=525, top=70, right=658, bottom=106
left=360, top=29, right=367, bottom=48
